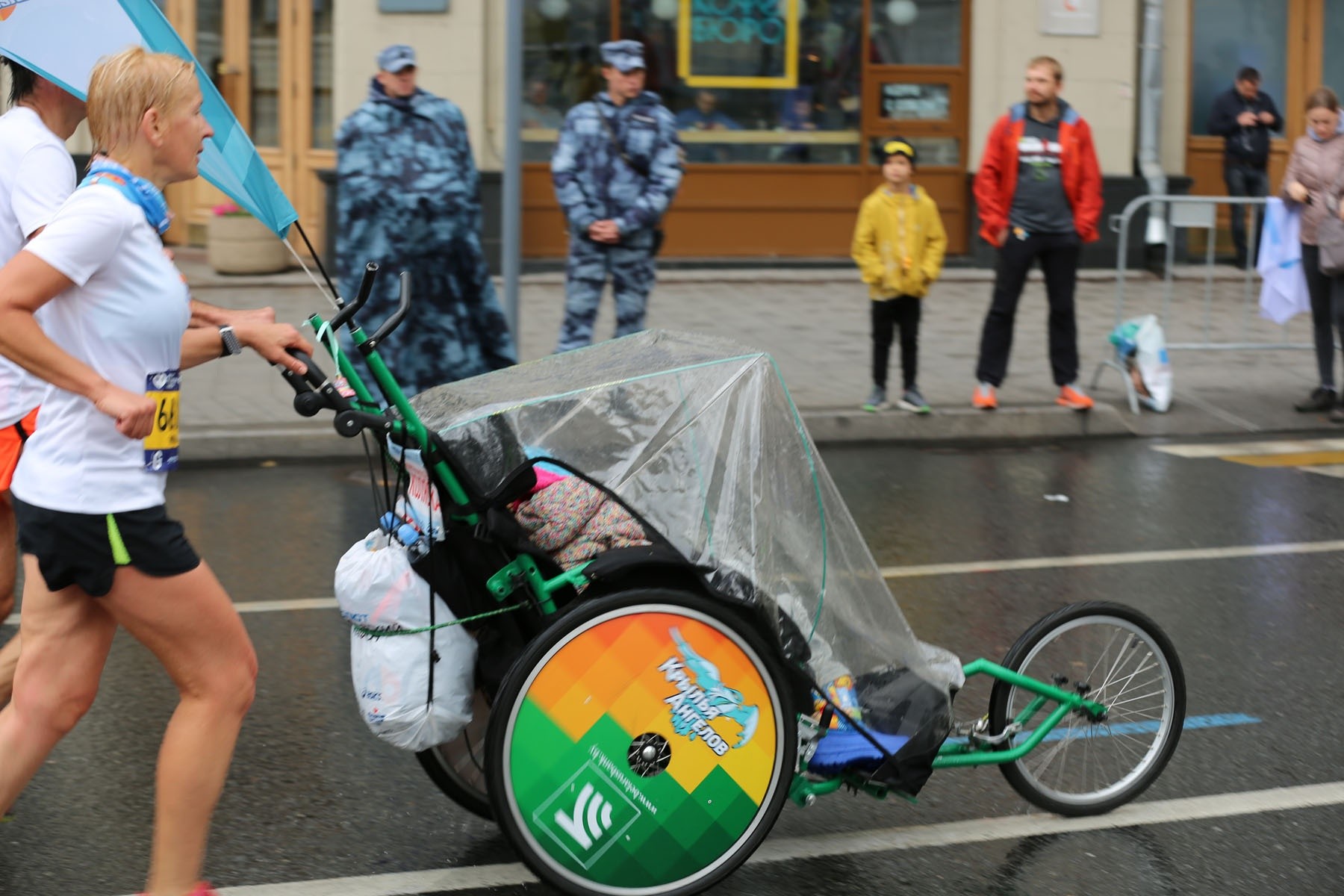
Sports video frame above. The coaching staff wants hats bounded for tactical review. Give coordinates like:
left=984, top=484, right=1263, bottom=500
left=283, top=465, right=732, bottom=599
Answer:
left=600, top=39, right=645, bottom=72
left=879, top=136, right=916, bottom=168
left=376, top=45, right=417, bottom=73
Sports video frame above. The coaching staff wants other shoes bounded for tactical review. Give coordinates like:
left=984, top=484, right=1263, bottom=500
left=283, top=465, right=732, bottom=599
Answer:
left=131, top=880, right=219, bottom=896
left=1327, top=391, right=1344, bottom=423
left=1293, top=388, right=1339, bottom=413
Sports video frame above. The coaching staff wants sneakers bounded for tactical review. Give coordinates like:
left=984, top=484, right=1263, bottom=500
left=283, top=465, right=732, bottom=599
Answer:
left=861, top=386, right=891, bottom=412
left=897, top=388, right=931, bottom=414
left=1055, top=384, right=1093, bottom=409
left=971, top=381, right=996, bottom=409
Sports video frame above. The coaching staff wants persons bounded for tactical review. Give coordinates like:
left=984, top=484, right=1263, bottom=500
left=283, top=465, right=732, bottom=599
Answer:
left=525, top=62, right=866, bottom=163
left=968, top=56, right=1105, bottom=410
left=851, top=135, right=947, bottom=411
left=329, top=43, right=513, bottom=409
left=1285, top=87, right=1344, bottom=421
left=550, top=39, right=682, bottom=352
left=1209, top=66, right=1284, bottom=268
left=0, top=51, right=313, bottom=896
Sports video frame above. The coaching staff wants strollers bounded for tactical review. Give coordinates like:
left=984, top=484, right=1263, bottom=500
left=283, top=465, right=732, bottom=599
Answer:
left=260, top=260, right=1191, bottom=896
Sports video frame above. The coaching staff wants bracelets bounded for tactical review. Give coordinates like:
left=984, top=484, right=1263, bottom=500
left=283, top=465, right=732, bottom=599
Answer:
left=216, top=324, right=244, bottom=359
